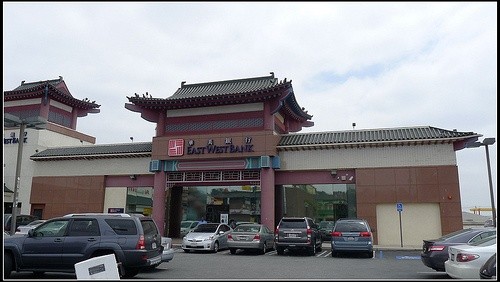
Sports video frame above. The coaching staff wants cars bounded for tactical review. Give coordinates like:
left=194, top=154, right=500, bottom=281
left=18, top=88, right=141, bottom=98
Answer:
left=182, top=223, right=234, bottom=253
left=180, top=220, right=200, bottom=236
left=420, top=226, right=497, bottom=279
left=329, top=218, right=376, bottom=258
left=315, top=220, right=335, bottom=241
left=4, top=213, right=175, bottom=271
left=226, top=224, right=274, bottom=255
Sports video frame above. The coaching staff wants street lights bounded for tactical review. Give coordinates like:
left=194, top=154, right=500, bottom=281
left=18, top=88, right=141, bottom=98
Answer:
left=4, top=113, right=47, bottom=236
left=466, top=137, right=496, bottom=228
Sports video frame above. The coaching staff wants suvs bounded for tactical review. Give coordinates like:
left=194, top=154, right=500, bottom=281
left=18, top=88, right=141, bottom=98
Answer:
left=274, top=216, right=325, bottom=256
left=5, top=215, right=164, bottom=279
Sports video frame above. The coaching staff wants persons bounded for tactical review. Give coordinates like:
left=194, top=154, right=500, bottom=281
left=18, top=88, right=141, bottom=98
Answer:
left=198, top=217, right=207, bottom=225
